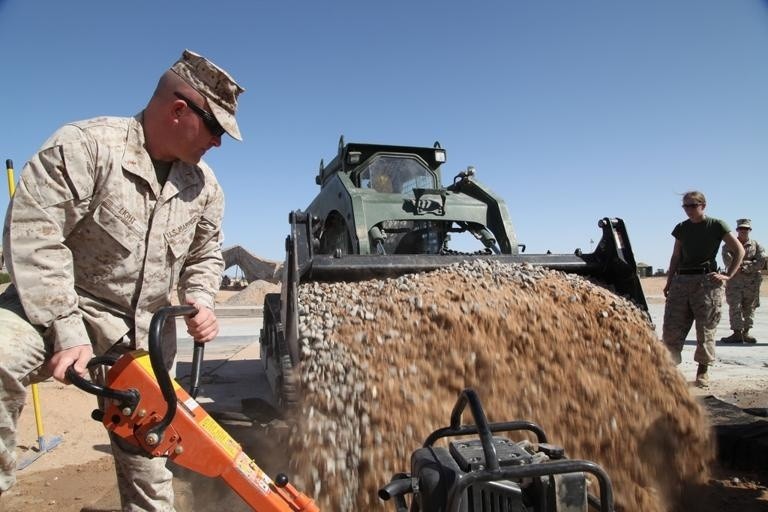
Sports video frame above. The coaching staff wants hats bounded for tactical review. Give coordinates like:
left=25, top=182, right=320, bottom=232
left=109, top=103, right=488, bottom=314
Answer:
left=169, top=48, right=245, bottom=143
left=737, top=218, right=752, bottom=230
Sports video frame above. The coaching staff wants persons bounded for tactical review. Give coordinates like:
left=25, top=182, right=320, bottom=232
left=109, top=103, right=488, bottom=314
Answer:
left=663, top=191, right=746, bottom=391
left=722, top=218, right=768, bottom=343
left=0, top=49, right=246, bottom=512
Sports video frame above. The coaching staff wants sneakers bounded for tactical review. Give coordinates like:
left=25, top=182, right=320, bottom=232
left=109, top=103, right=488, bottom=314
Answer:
left=695, top=363, right=710, bottom=388
left=720, top=329, right=757, bottom=344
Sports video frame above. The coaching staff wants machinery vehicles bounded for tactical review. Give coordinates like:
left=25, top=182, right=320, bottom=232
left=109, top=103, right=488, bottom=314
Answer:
left=259, top=135, right=664, bottom=450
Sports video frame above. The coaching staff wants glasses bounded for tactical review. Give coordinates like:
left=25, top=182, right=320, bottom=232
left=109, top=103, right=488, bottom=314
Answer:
left=172, top=90, right=227, bottom=137
left=682, top=203, right=702, bottom=209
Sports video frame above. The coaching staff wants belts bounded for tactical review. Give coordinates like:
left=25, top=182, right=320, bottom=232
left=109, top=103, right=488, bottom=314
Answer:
left=679, top=267, right=717, bottom=275
